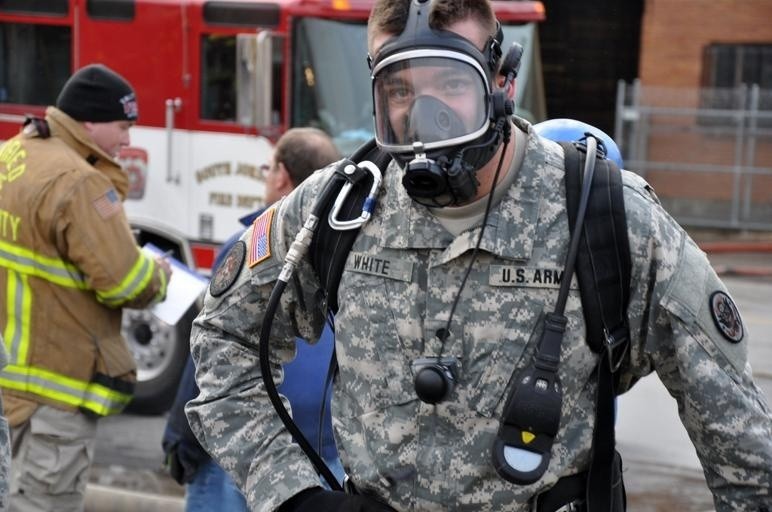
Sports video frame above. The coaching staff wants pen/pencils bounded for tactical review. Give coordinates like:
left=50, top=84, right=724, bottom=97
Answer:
left=160, top=250, right=173, bottom=261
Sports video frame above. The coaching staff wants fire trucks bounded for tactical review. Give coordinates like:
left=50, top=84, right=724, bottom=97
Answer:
left=1, top=0, right=555, bottom=418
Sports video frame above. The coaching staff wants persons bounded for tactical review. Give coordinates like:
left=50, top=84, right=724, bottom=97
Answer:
left=0, top=62, right=172, bottom=512
left=184, top=0, right=772, bottom=512
left=161, top=127, right=347, bottom=512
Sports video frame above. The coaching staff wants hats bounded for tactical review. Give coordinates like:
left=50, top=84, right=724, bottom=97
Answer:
left=56, top=63, right=138, bottom=122
